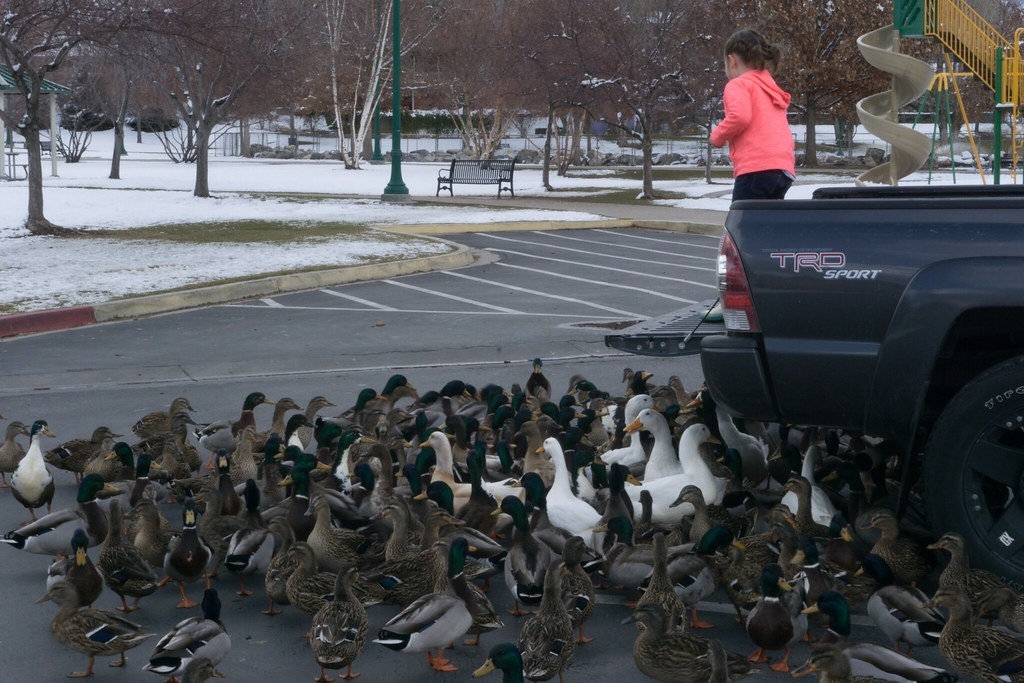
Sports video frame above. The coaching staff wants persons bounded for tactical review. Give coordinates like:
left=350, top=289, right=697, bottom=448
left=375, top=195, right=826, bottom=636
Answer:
left=697, top=28, right=795, bottom=322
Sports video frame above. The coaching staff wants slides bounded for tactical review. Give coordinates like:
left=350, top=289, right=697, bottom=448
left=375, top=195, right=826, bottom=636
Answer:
left=855, top=24, right=933, bottom=187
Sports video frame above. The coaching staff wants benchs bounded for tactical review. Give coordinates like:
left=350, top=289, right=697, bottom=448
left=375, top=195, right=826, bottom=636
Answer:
left=435, top=156, right=519, bottom=200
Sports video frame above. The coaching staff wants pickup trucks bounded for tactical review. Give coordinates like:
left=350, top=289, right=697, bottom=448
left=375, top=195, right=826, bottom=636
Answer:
left=604, top=186, right=1023, bottom=593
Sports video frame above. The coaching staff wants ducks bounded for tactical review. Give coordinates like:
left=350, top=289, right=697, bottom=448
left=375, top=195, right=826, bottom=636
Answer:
left=0, top=358, right=1024, bottom=683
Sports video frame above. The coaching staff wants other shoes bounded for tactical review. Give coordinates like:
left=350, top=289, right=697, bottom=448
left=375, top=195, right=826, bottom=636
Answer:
left=701, top=300, right=725, bottom=323
